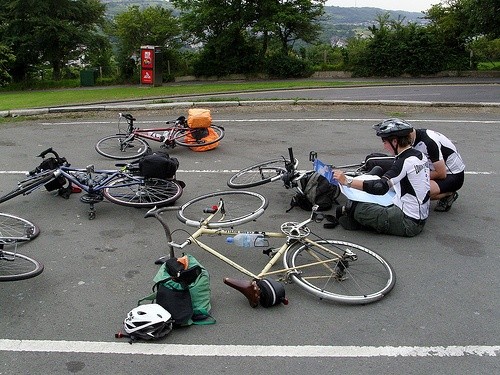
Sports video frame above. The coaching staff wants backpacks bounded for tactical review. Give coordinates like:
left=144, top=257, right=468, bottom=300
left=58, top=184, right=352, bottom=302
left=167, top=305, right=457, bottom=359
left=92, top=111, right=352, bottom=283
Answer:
left=138, top=151, right=180, bottom=178
left=138, top=252, right=216, bottom=328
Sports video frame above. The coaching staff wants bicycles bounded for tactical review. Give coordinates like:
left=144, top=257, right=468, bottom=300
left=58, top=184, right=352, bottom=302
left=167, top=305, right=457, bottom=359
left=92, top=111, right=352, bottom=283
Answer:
left=0, top=212, right=44, bottom=282
left=144, top=189, right=398, bottom=310
left=227, top=146, right=366, bottom=213
left=0, top=146, right=186, bottom=220
left=95, top=113, right=226, bottom=160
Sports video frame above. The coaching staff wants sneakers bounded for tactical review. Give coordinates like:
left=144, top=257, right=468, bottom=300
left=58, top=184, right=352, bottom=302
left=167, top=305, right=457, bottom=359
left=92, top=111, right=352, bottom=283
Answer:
left=434, top=192, right=459, bottom=212
left=337, top=216, right=365, bottom=231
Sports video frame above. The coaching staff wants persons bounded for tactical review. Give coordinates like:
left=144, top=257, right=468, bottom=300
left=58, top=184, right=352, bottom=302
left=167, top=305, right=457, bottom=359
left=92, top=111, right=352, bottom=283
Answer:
left=333, top=118, right=430, bottom=237
left=409, top=124, right=465, bottom=211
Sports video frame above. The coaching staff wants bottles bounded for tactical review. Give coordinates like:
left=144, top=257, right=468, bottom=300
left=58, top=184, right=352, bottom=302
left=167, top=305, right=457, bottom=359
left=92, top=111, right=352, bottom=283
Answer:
left=263, top=247, right=279, bottom=255
left=93, top=172, right=108, bottom=185
left=227, top=234, right=264, bottom=248
left=147, top=132, right=161, bottom=138
left=163, top=131, right=169, bottom=138
left=75, top=171, right=89, bottom=183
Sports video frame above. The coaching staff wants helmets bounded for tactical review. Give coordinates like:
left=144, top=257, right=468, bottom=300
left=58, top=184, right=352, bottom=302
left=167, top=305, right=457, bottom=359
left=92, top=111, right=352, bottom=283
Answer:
left=123, top=303, right=176, bottom=345
left=372, top=117, right=413, bottom=155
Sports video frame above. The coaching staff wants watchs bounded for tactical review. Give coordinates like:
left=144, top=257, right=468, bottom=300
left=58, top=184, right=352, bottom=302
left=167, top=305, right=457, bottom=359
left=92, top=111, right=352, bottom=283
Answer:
left=346, top=178, right=353, bottom=188
